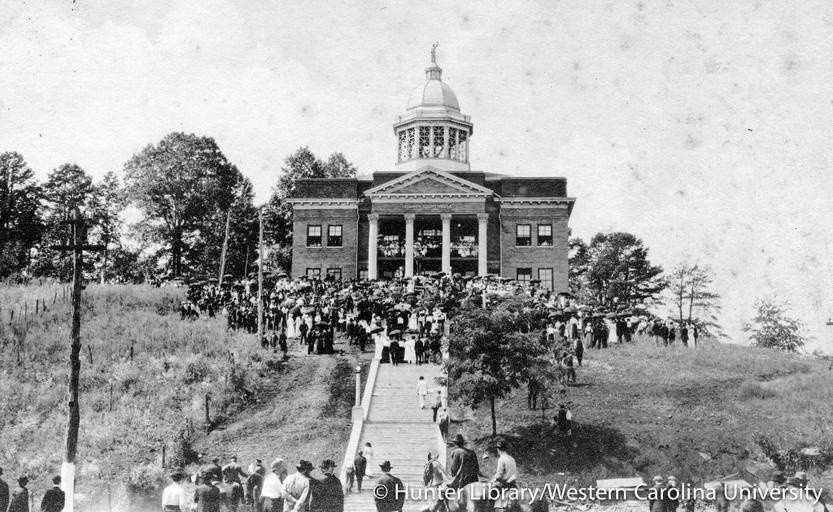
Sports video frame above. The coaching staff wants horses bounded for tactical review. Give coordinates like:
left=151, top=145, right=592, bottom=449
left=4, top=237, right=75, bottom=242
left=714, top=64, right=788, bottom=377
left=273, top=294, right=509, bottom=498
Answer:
left=422, top=452, right=519, bottom=512
left=477, top=471, right=549, bottom=512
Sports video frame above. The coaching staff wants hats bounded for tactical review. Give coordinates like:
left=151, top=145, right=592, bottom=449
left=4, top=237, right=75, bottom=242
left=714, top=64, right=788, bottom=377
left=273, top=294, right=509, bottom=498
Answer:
left=17, top=475, right=30, bottom=483
left=212, top=456, right=261, bottom=463
left=452, top=434, right=467, bottom=444
left=318, top=459, right=338, bottom=468
left=494, top=439, right=510, bottom=449
left=379, top=459, right=394, bottom=469
left=652, top=474, right=677, bottom=482
left=268, top=461, right=283, bottom=472
left=168, top=467, right=213, bottom=479
left=294, top=459, right=316, bottom=469
left=771, top=469, right=810, bottom=484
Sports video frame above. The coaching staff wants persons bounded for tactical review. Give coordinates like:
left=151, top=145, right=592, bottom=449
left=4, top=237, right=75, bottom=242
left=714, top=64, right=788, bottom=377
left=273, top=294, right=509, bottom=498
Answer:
left=565, top=350, right=577, bottom=382
left=0, top=466, right=9, bottom=512
left=353, top=449, right=367, bottom=494
left=160, top=456, right=343, bottom=511
left=375, top=235, right=478, bottom=258
left=557, top=404, right=572, bottom=437
left=372, top=459, right=405, bottom=512
left=552, top=416, right=560, bottom=434
left=526, top=373, right=539, bottom=408
left=361, top=441, right=372, bottom=479
left=645, top=469, right=829, bottom=512
left=429, top=390, right=442, bottom=421
left=40, top=474, right=65, bottom=512
left=415, top=375, right=432, bottom=408
left=486, top=440, right=516, bottom=511
left=437, top=407, right=448, bottom=438
left=342, top=451, right=355, bottom=494
left=4, top=474, right=29, bottom=512
left=421, top=434, right=478, bottom=512
left=429, top=40, right=438, bottom=66
